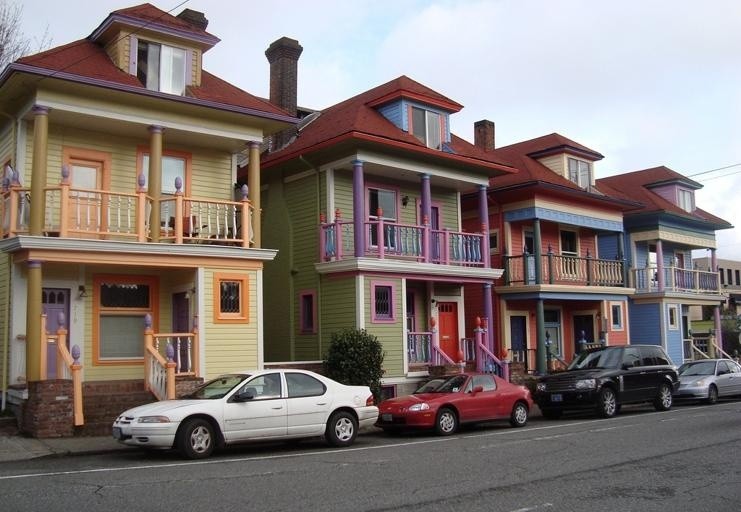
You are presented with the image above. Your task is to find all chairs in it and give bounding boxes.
[168,210,242,247]
[246,387,257,395]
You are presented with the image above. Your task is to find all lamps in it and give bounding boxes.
[402,195,409,207]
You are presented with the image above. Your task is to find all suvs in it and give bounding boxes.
[533,344,681,418]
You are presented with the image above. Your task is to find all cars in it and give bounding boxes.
[112,369,380,459]
[374,372,534,437]
[672,359,741,405]
